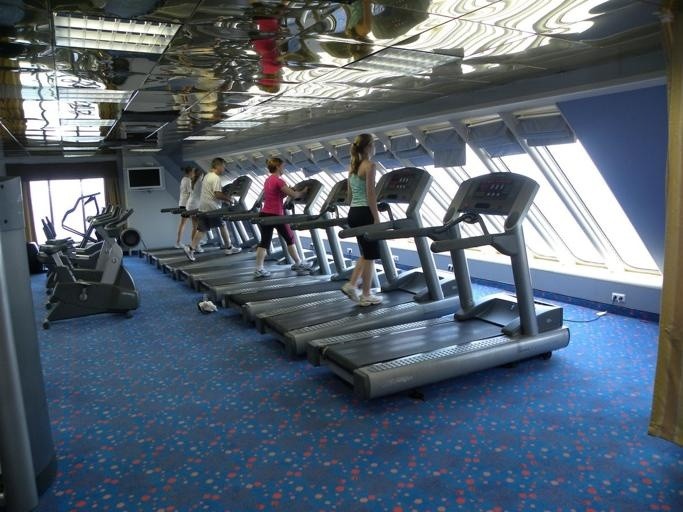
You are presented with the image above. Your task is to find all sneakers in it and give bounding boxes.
[361,294,383,306]
[342,283,360,301]
[255,270,270,277]
[296,261,314,271]
[174,241,205,261]
[224,245,242,254]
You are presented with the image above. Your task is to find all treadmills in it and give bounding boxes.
[307,173,571,401]
[140,176,353,319]
[254,168,466,357]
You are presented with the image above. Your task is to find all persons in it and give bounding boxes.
[251,156,314,279]
[157,0,393,133]
[187,168,207,257]
[175,162,192,251]
[184,158,241,261]
[340,133,384,307]
